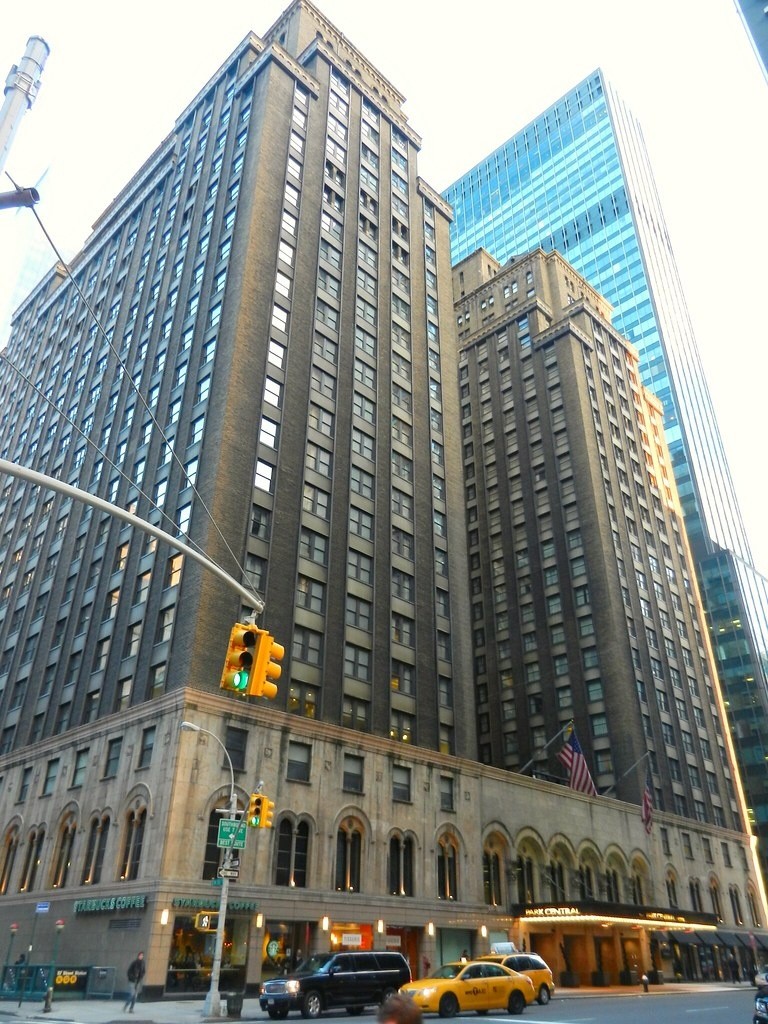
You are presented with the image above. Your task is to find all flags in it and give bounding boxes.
[554,727,598,796]
[642,754,653,834]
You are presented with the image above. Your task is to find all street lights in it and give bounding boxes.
[181,721,238,1018]
[43,920,64,1012]
[0,924,18,991]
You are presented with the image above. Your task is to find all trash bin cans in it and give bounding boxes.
[227,995,243,1018]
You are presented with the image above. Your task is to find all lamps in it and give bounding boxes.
[161,908,487,938]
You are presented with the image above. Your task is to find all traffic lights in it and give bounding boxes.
[247,635,284,701]
[220,624,253,695]
[194,913,212,931]
[248,795,262,828]
[259,797,276,829]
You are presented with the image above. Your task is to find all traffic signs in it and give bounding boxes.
[217,867,239,878]
[223,860,241,868]
[217,819,247,848]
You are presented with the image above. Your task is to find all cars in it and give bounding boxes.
[751,965,768,1024]
[398,959,536,1018]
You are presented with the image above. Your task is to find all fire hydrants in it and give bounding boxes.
[642,975,650,992]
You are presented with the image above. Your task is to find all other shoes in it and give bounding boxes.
[123,1007,134,1014]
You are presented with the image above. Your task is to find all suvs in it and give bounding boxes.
[258,950,412,1020]
[473,942,555,1004]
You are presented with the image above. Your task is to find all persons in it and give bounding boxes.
[460,949,469,961]
[123,951,146,1013]
[728,953,741,984]
[166,945,291,991]
[291,949,304,972]
[15,954,28,990]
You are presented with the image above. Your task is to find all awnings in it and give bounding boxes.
[651,929,768,951]
